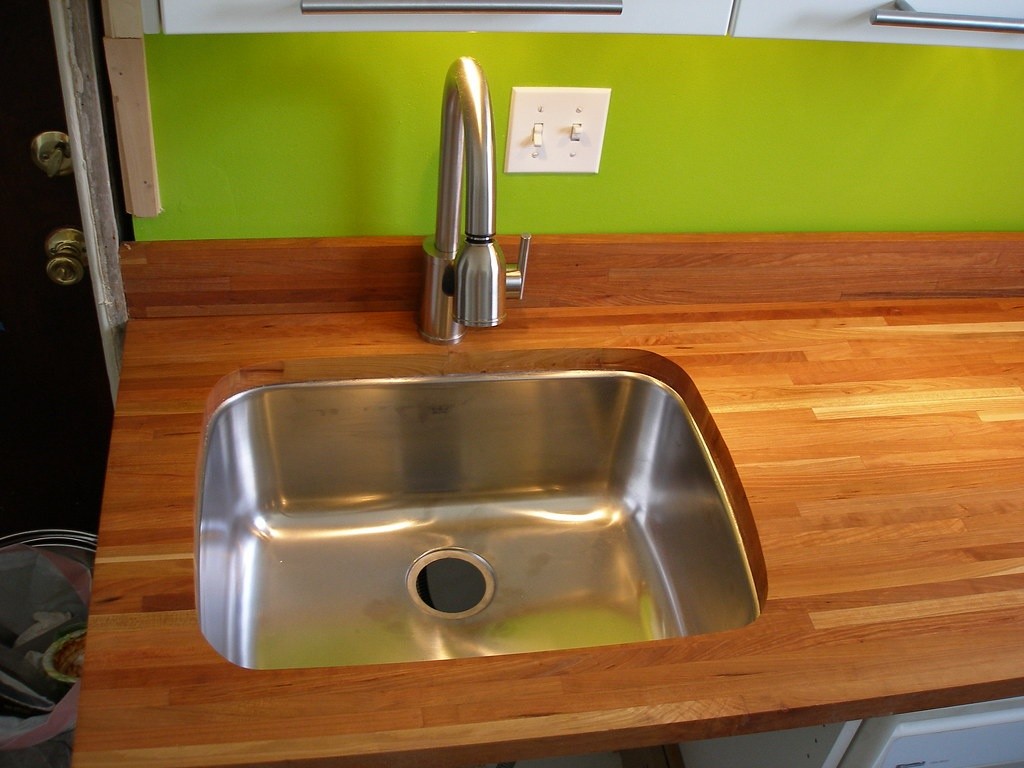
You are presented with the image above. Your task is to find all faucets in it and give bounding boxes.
[419,56,532,347]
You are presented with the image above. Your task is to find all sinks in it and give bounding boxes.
[193,345,769,671]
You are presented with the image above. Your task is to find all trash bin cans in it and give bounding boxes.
[0,529,98,768]
